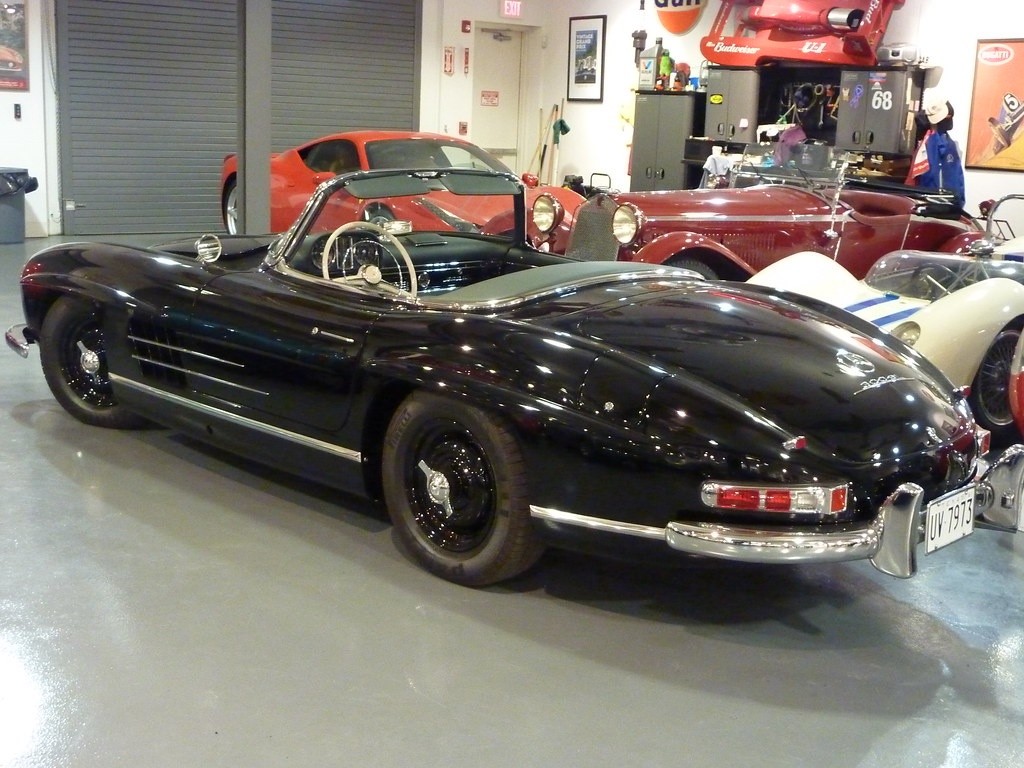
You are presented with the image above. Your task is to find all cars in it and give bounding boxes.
[221,131,590,254]
[564,143,985,280]
[4,167,994,591]
[743,232,1024,533]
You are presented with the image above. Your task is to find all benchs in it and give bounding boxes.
[436,259,684,301]
[818,188,916,215]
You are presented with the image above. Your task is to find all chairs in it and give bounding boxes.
[308,141,446,175]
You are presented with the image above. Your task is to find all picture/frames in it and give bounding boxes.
[567,14,608,100]
[963,37,1024,171]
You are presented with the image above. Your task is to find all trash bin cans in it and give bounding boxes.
[0,167,28,245]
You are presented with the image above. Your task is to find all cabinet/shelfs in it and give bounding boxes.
[836,66,927,158]
[706,63,770,144]
[629,88,704,193]
